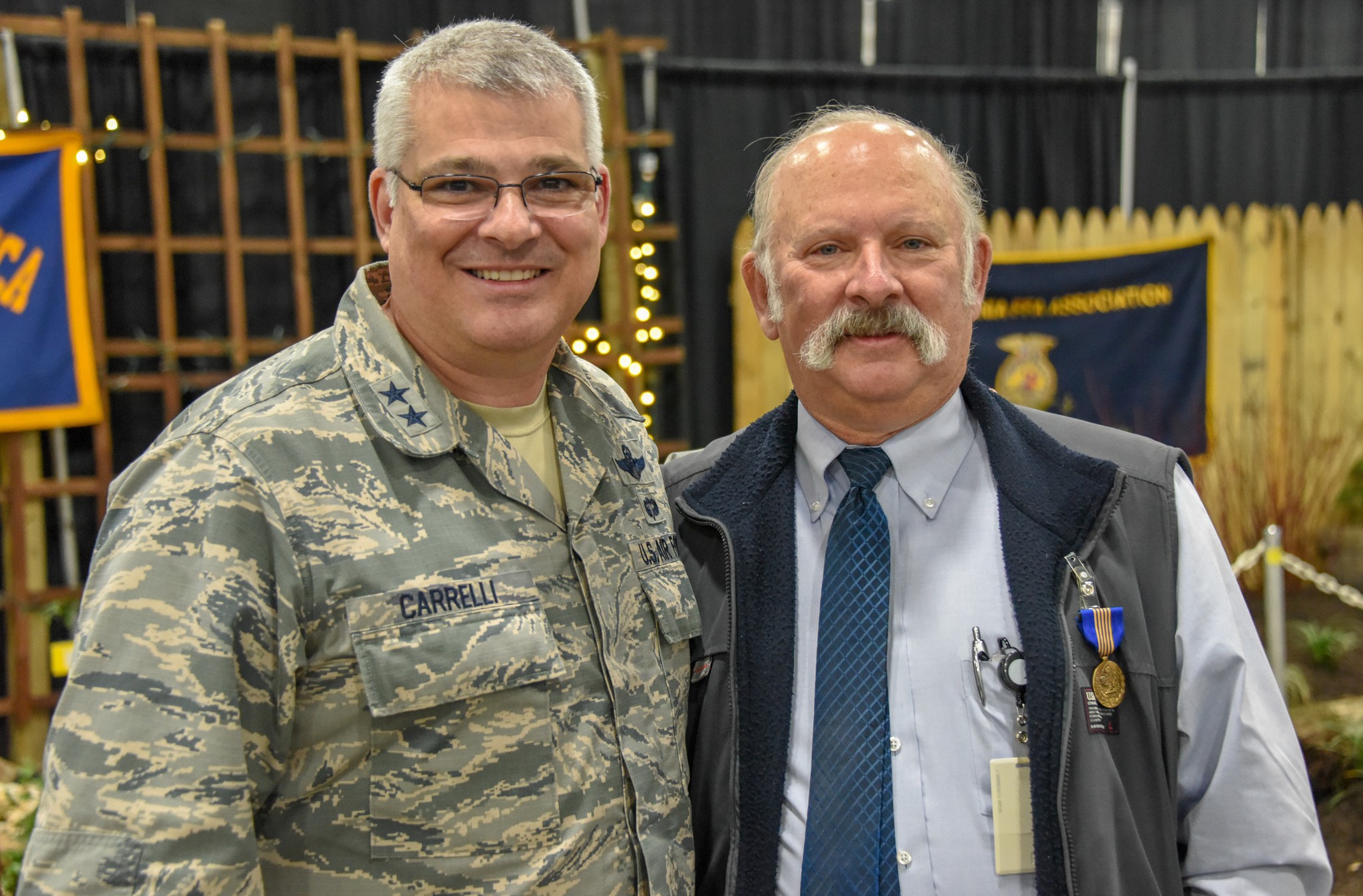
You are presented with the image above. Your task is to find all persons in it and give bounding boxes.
[655,100,1343,896]
[10,17,707,896]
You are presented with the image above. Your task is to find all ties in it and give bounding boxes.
[798,445,902,896]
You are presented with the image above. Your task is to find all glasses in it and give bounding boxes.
[385,167,604,218]
